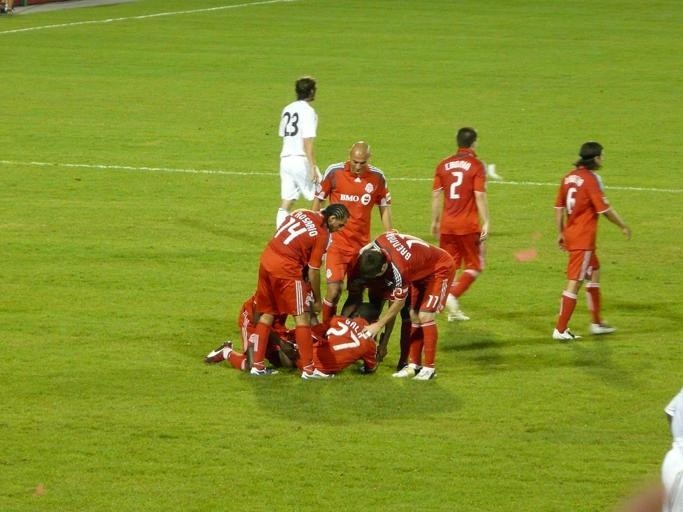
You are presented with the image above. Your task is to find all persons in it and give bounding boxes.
[429,127,491,324]
[205,204,457,383]
[309,140,394,324]
[612,384,683,512]
[274,76,325,233]
[551,139,632,341]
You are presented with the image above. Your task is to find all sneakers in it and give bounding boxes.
[203,341,232,363]
[552,327,580,340]
[413,367,435,381]
[300,368,334,381]
[447,312,469,322]
[392,363,421,378]
[250,367,278,376]
[591,323,614,334]
